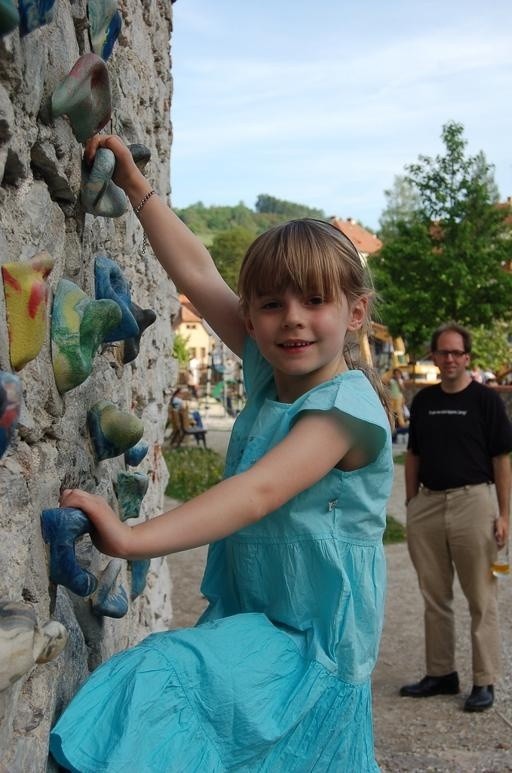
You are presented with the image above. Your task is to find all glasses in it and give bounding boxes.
[434,349,467,357]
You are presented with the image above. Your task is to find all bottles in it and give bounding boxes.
[492,540,510,576]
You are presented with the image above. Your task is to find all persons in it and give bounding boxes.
[399,327,512,713]
[49,131,395,773]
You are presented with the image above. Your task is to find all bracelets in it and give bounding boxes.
[135,190,156,217]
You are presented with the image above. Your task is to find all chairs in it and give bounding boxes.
[167,408,200,446]
[176,406,207,449]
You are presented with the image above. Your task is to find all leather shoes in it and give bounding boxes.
[464,683,493,712]
[399,670,459,697]
